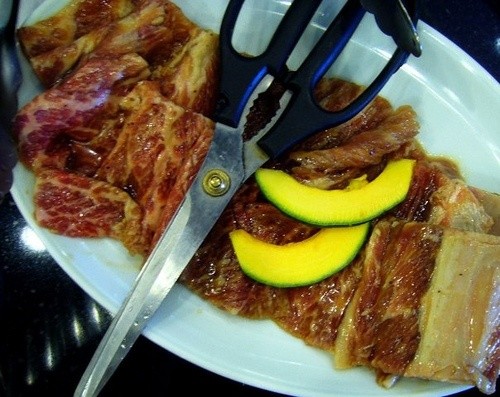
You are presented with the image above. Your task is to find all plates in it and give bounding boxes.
[8,0,499,395]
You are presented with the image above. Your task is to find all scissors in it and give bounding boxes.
[73,0,422,397]
